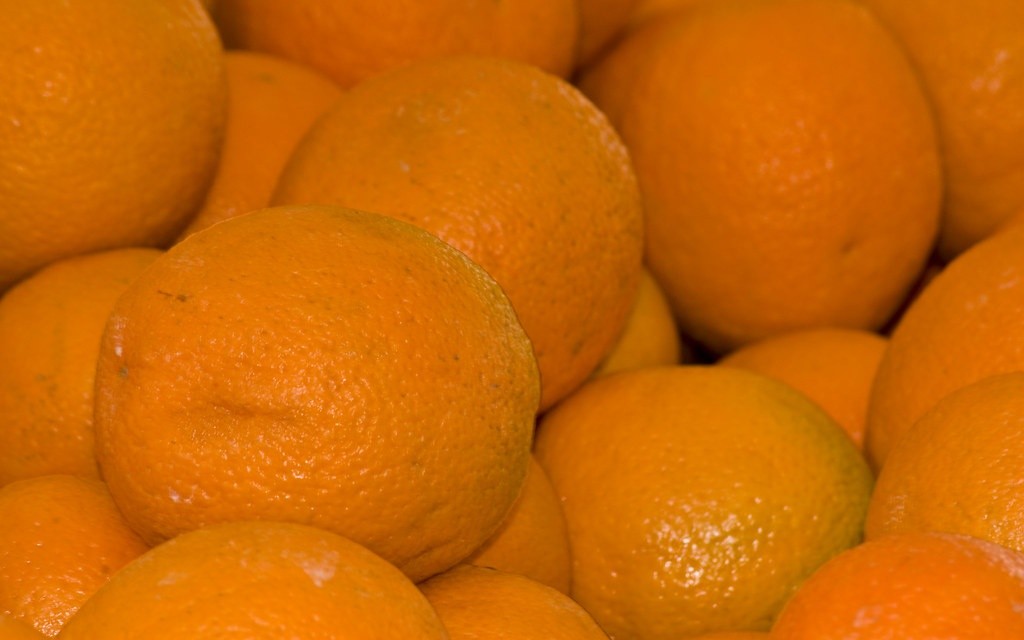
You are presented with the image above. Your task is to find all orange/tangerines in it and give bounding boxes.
[0,0,1024,640]
